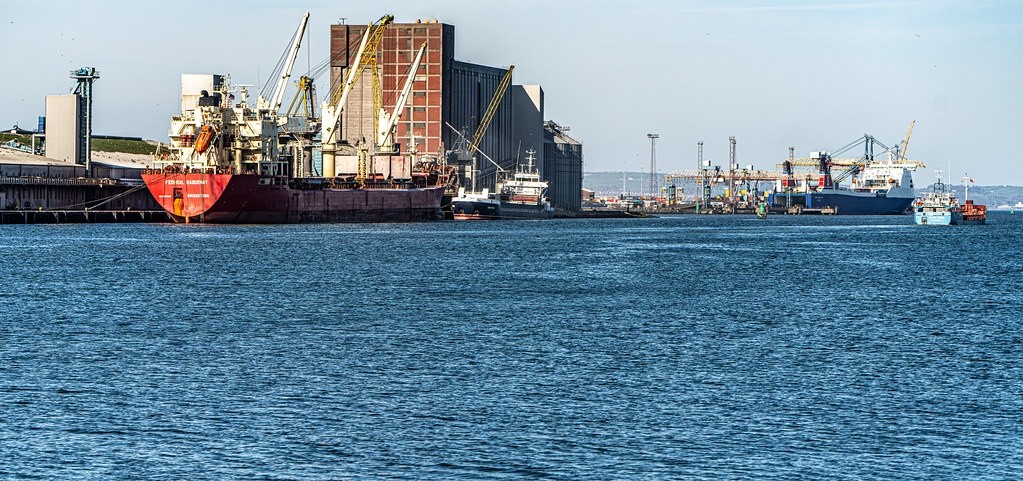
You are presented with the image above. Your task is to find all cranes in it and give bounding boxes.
[777,119,927,169]
[466,63,517,156]
[292,11,427,181]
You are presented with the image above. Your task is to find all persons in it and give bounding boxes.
[146,165,255,174]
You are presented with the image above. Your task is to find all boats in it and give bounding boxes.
[194,125,215,152]
[451,140,555,221]
[139,74,447,223]
[910,161,987,226]
[767,153,918,216]
